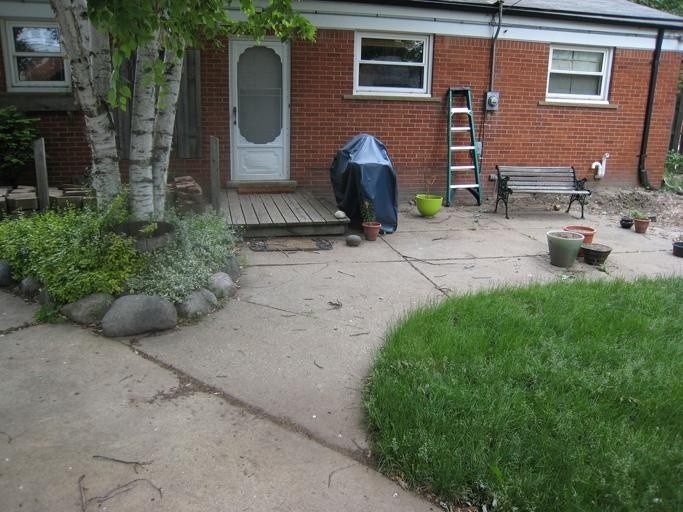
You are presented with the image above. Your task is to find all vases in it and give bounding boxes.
[546,226,612,268]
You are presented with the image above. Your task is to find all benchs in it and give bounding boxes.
[495,165,591,219]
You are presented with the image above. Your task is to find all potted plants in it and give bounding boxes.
[416,175,443,218]
[632,209,651,233]
[620,216,633,229]
[360,199,381,241]
[672,234,683,257]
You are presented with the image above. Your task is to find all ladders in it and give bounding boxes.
[447,87,482,206]
